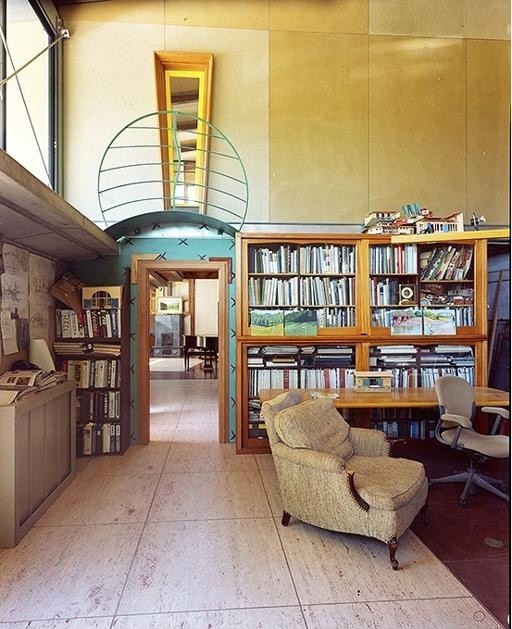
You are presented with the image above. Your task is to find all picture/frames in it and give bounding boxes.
[156,296,183,314]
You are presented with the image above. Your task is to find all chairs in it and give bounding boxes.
[260,389,429,570]
[427,376,510,507]
[183,336,218,368]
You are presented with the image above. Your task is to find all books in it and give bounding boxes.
[369,408,440,440]
[52,302,122,458]
[370,307,474,336]
[353,345,475,392]
[419,245,473,305]
[248,399,268,439]
[0,363,68,408]
[248,307,356,337]
[247,245,355,275]
[248,277,355,306]
[247,345,356,398]
[369,245,417,305]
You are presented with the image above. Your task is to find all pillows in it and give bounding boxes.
[274,398,354,461]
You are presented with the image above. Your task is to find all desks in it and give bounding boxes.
[0,379,76,548]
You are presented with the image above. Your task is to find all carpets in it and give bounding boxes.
[148,358,204,371]
[386,437,509,629]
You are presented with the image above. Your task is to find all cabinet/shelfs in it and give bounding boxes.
[55,267,130,456]
[235,231,487,453]
